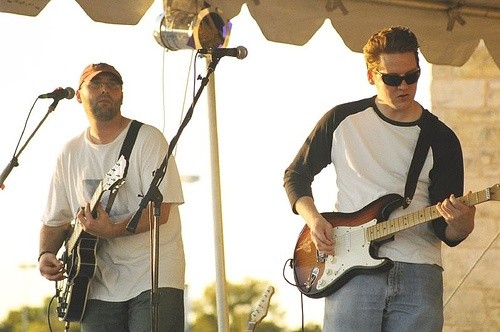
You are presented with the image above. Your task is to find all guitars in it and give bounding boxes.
[246,286,275,332]
[47,153,129,332]
[282,184,497,298]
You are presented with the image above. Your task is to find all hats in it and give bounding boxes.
[78,62,122,88]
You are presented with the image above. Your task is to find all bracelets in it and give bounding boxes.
[38,250,52,262]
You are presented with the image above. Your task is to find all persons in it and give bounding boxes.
[283,26,476,332]
[38,63,185,332]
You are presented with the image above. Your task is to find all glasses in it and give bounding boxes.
[372,65,421,87]
[86,80,121,90]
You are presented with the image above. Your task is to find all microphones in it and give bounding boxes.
[198,46,248,59]
[39,87,75,99]
